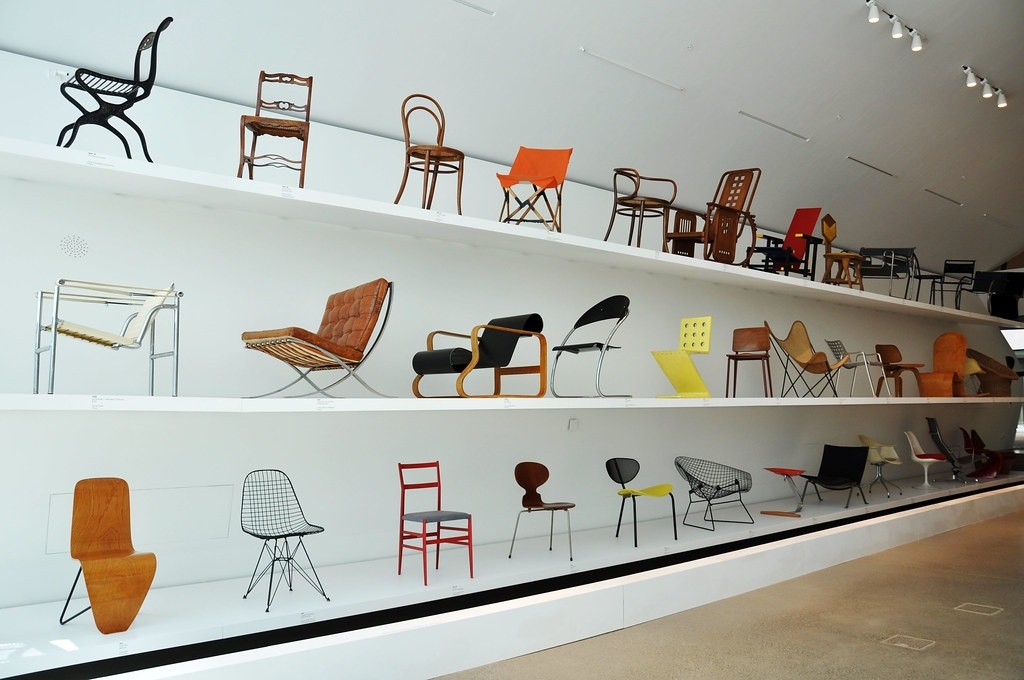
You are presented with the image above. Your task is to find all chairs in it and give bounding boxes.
[57,17,173,162]
[33,279,183,394]
[605,458,677,548]
[412,313,547,398]
[663,168,762,267]
[509,461,576,561]
[761,417,1024,517]
[726,320,1019,397]
[60,477,157,635]
[605,168,676,253]
[674,455,754,531]
[822,213,1024,317]
[398,461,473,586]
[551,294,630,397]
[237,70,313,189]
[393,94,465,216]
[746,207,823,281]
[240,469,331,612]
[242,278,399,400]
[496,146,574,232]
[651,316,712,397]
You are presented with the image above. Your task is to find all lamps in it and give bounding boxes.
[865,0,926,51]
[963,65,1007,108]
